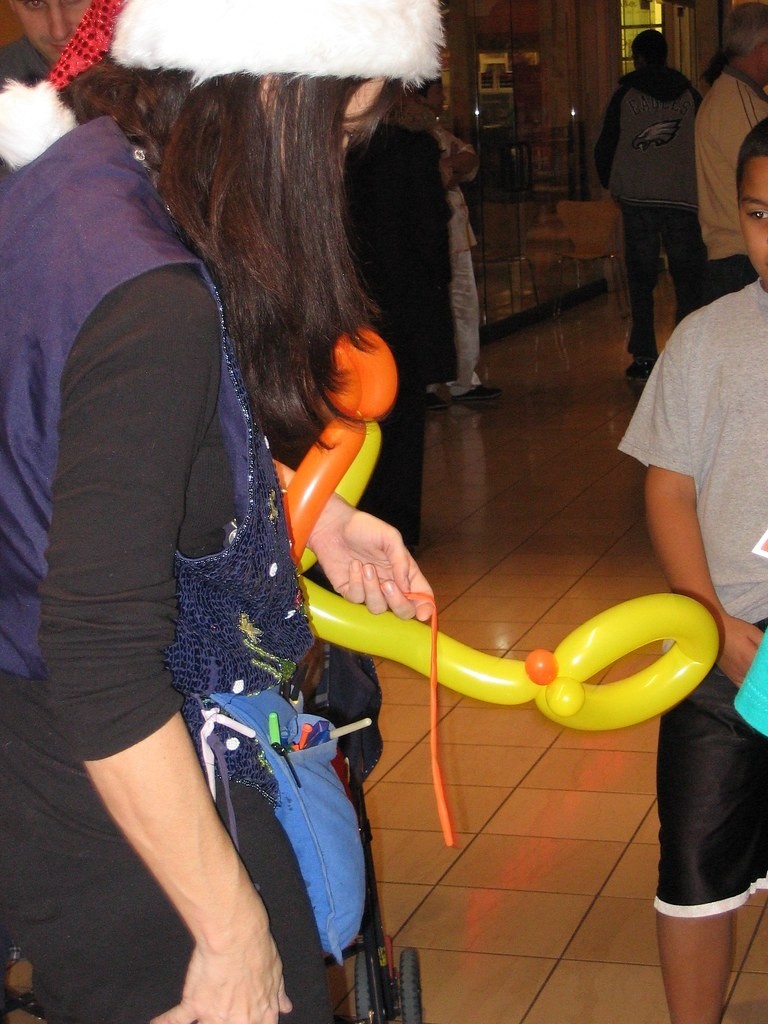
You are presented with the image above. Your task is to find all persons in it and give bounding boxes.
[0,0,768,1024]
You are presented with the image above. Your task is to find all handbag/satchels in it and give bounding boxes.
[209,688,366,964]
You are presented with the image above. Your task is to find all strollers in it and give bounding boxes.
[297,629,424,1024]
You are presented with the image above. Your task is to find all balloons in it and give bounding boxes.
[268,329,398,567]
[293,418,718,732]
[397,593,455,848]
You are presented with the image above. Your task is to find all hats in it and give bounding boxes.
[0,0,446,172]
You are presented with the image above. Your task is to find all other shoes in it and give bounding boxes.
[625,358,656,382]
[451,385,503,400]
[425,392,449,409]
